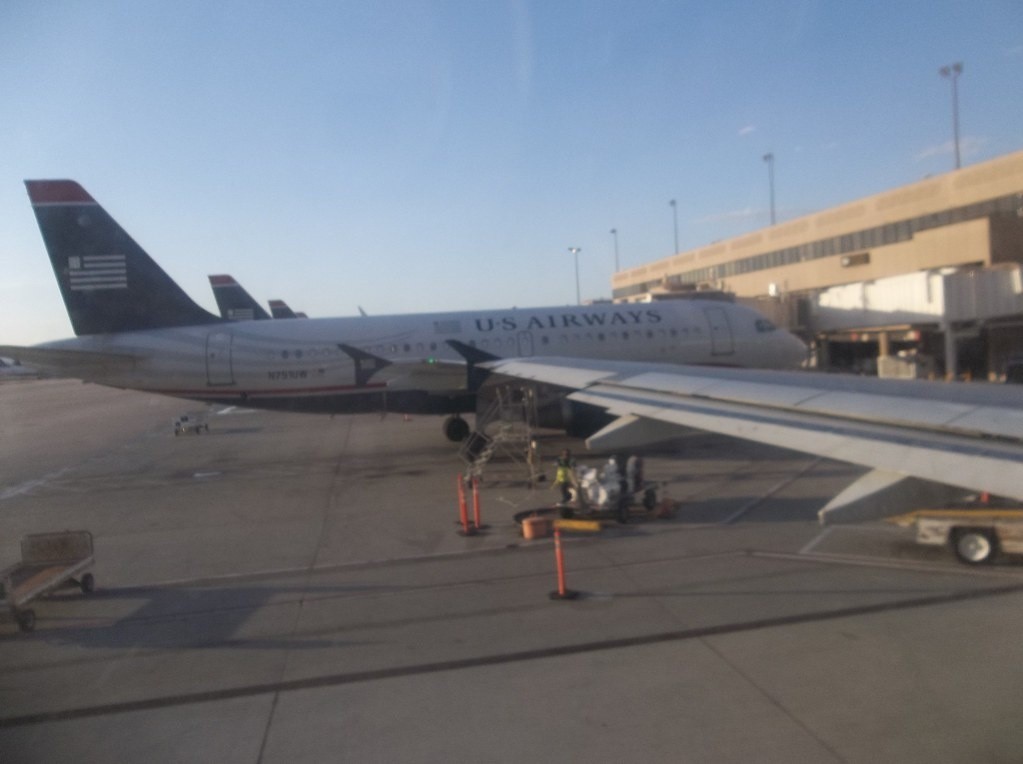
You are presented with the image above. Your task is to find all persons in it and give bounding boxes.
[550,449,578,503]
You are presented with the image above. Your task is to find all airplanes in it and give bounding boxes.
[1,177,1021,529]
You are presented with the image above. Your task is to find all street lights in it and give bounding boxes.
[758,150,778,228]
[940,62,964,174]
[608,229,620,272]
[567,247,581,307]
[668,199,678,258]
